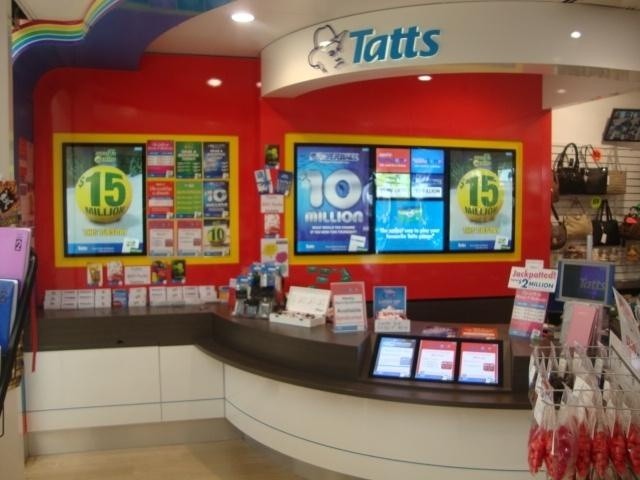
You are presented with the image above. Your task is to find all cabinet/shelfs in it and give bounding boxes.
[0,250,38,413]
[551,144,640,282]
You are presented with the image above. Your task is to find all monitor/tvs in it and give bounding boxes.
[368,334,505,390]
[553,258,614,308]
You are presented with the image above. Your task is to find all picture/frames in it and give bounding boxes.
[603,106,640,142]
